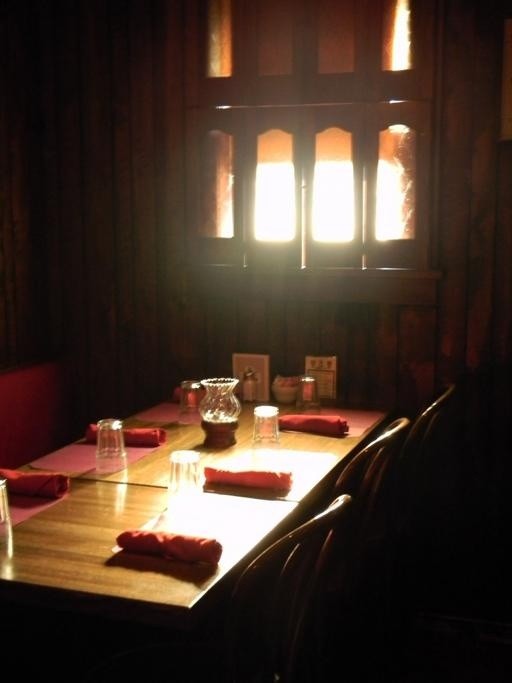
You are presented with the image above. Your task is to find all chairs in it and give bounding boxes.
[398,384,455,476]
[88,494,352,683]
[331,417,408,505]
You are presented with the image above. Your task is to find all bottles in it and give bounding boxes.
[92,417,128,474]
[297,376,319,402]
[241,368,256,403]
[175,380,202,414]
[246,403,281,444]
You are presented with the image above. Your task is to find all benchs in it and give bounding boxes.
[0,359,78,468]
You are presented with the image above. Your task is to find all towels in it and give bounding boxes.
[173,388,204,403]
[87,424,167,445]
[206,466,291,489]
[117,532,222,565]
[278,415,350,436]
[0,467,69,498]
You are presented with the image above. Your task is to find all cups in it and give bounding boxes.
[167,451,204,516]
[0,479,15,546]
